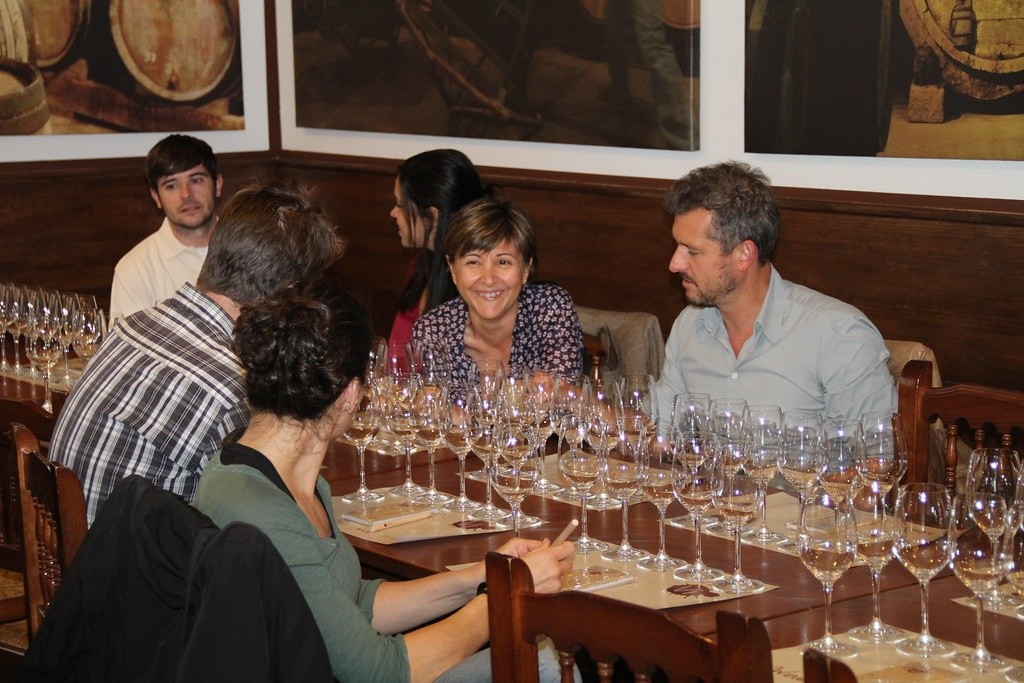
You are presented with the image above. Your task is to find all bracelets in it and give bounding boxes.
[477,581,488,595]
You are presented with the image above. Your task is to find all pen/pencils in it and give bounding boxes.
[551,519,579,547]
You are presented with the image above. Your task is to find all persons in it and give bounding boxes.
[598,1,700,150]
[189,279,578,683]
[108,133,224,334]
[414,197,583,410]
[388,148,494,387]
[535,159,898,483]
[47,172,346,529]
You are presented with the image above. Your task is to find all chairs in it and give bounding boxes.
[482,549,778,681]
[572,303,663,406]
[9,423,91,651]
[898,359,1024,490]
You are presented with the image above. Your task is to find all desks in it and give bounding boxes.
[1,285,1024,682]
